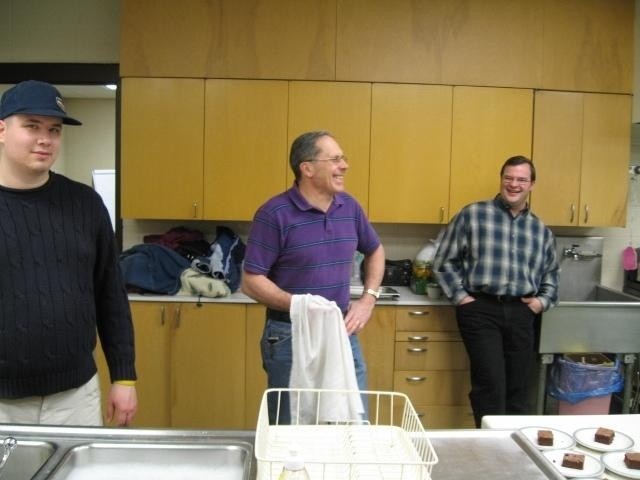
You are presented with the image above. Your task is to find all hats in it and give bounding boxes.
[0,80,82,125]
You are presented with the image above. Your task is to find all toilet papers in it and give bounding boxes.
[599,237,628,291]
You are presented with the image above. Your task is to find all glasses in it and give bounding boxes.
[503,176,531,183]
[302,155,345,162]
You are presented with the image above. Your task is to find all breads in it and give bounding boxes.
[537,430,554,446]
[595,426,615,444]
[562,453,585,470]
[625,452,639,468]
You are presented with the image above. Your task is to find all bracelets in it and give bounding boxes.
[111,380,137,386]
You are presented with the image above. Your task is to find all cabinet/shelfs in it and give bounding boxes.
[449,86,534,224]
[91,302,245,430]
[121,77,204,220]
[245,304,395,430]
[529,90,634,227]
[204,79,288,221]
[395,306,478,428]
[368,83,451,224]
[288,80,369,224]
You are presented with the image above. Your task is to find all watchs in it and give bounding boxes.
[363,288,380,300]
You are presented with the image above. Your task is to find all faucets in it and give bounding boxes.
[564,245,579,261]
[0,437,17,474]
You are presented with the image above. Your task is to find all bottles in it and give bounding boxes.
[410,263,438,294]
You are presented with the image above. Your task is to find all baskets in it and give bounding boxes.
[359,259,413,286]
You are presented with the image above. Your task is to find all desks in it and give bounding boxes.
[481,414,640,479]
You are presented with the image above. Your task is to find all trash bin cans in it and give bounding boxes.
[555,352,619,415]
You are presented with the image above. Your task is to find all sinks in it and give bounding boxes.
[0,440,57,480]
[46,441,252,480]
[538,284,640,355]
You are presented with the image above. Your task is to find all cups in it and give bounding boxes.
[426,284,443,299]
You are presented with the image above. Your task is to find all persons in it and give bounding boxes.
[433,155,560,429]
[241,130,386,426]
[0,79,138,427]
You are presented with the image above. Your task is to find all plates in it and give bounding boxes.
[520,427,640,480]
